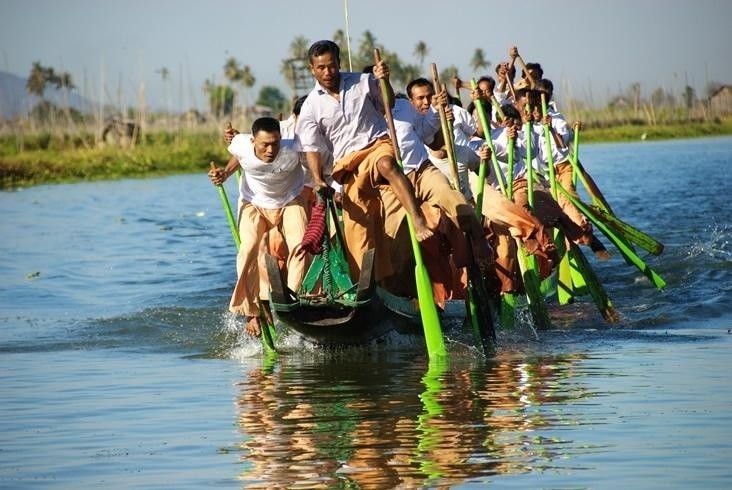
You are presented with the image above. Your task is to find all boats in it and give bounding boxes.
[264,248,557,349]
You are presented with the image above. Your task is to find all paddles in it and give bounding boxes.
[210,140,278,356]
[373,45,448,358]
[430,46,667,344]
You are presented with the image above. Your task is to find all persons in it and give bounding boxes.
[295,40,433,287]
[362,44,595,310]
[208,96,344,337]
